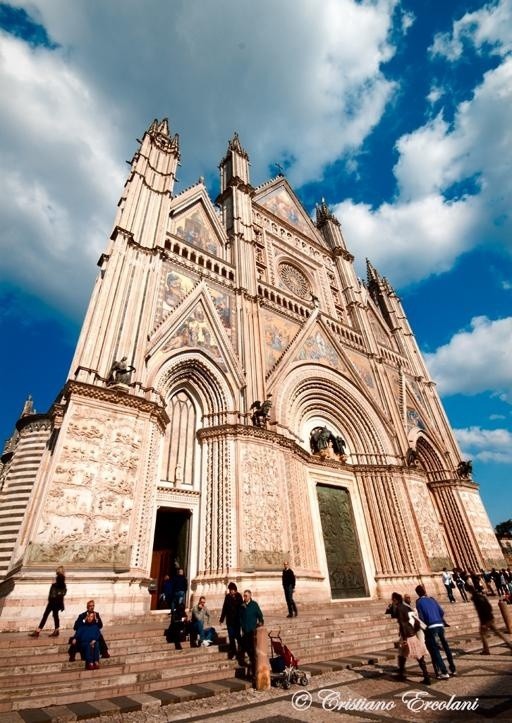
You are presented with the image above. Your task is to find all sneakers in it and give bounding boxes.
[452,670,458,676]
[436,673,450,679]
[201,639,213,647]
[85,661,100,670]
[420,679,431,685]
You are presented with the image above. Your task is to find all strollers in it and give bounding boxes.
[267,629,310,689]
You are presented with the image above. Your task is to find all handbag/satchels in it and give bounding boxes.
[268,655,287,673]
[449,578,456,588]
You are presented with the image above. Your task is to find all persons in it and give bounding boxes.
[28,565,68,637]
[439,564,512,603]
[112,356,130,384]
[168,567,188,615]
[156,574,171,607]
[72,611,101,671]
[66,599,110,661]
[282,560,299,618]
[167,603,201,650]
[403,594,413,607]
[415,585,456,678]
[388,592,431,685]
[192,597,215,647]
[238,588,264,666]
[465,584,512,654]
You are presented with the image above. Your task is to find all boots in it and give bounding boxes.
[27,630,40,637]
[48,629,59,637]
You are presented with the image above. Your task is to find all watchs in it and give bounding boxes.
[218,583,243,660]
